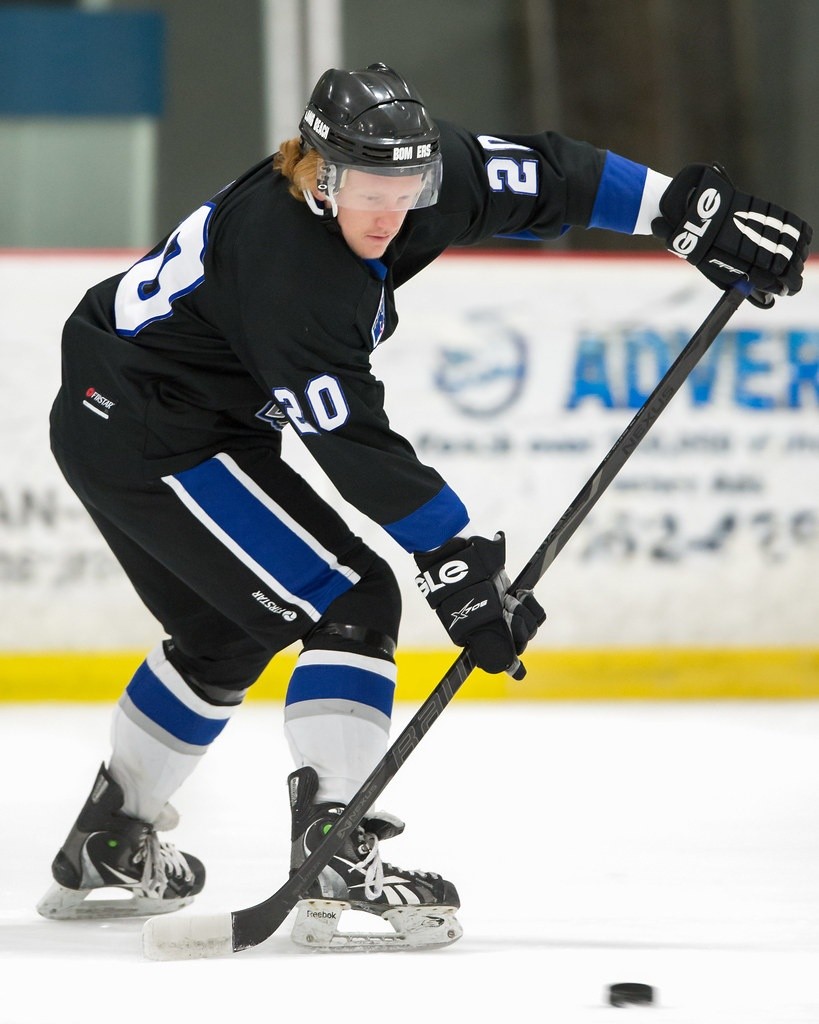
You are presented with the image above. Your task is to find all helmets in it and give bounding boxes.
[299,62,442,217]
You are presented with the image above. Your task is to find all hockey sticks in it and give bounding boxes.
[139,275,756,966]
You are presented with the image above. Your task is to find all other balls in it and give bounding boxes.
[608,983,654,1008]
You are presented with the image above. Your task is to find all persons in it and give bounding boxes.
[38,64,811,953]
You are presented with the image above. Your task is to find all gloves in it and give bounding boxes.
[651,161,813,309]
[414,530,546,681]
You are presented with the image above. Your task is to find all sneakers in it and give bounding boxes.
[288,766,463,953]
[36,761,206,920]
